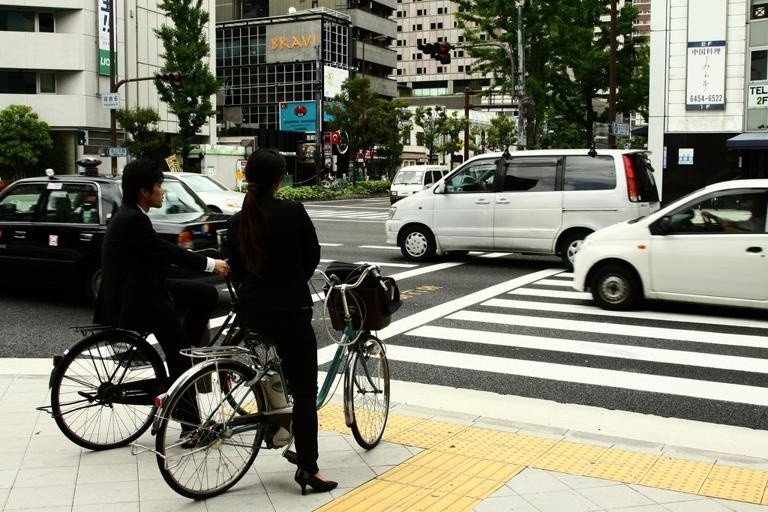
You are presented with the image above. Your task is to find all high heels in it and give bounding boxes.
[295,469,337,495]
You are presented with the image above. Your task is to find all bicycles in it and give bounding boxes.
[35,247,294,451]
[130,262,403,500]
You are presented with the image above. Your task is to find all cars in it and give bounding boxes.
[0,167,235,310]
[118,168,248,217]
[572,179,768,311]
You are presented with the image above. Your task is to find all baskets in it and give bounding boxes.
[322,280,392,331]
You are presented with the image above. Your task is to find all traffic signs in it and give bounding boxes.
[99,147,129,157]
[612,120,631,136]
[101,92,121,108]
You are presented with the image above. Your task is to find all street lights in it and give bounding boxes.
[362,36,386,181]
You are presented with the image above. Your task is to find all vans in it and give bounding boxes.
[390,164,450,206]
[385,141,661,272]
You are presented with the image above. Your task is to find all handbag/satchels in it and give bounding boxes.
[324,262,403,330]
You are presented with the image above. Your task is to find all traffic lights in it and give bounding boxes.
[434,52,451,64]
[77,131,86,145]
[155,71,183,82]
[413,40,451,53]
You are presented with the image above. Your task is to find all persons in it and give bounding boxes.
[699,197,766,232]
[92,158,230,449]
[229,145,338,494]
